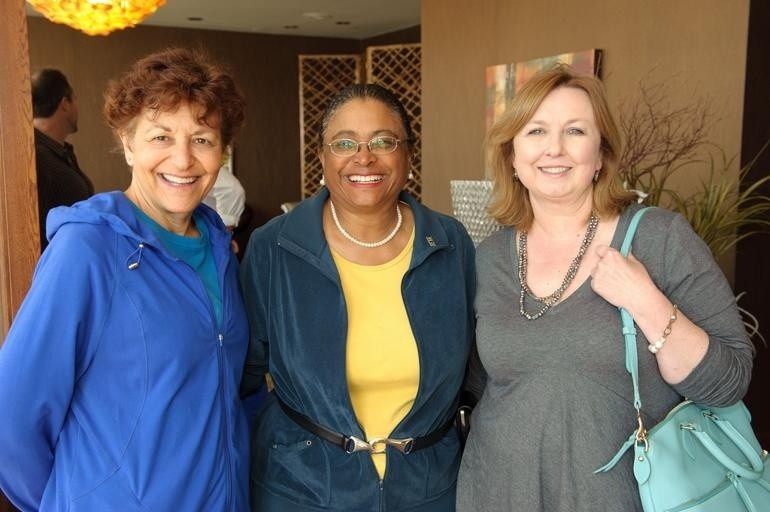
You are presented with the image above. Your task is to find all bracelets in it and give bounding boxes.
[644,303,680,353]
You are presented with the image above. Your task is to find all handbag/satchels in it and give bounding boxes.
[633,401,770,512]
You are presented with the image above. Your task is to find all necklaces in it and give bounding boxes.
[513,209,599,321]
[329,199,403,248]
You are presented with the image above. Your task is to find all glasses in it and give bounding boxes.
[319,135,411,156]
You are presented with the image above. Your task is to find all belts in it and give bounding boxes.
[272,388,459,456]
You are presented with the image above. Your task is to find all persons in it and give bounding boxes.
[0,47,261,512]
[447,63,753,512]
[221,79,491,511]
[27,66,95,240]
[193,157,249,257]
